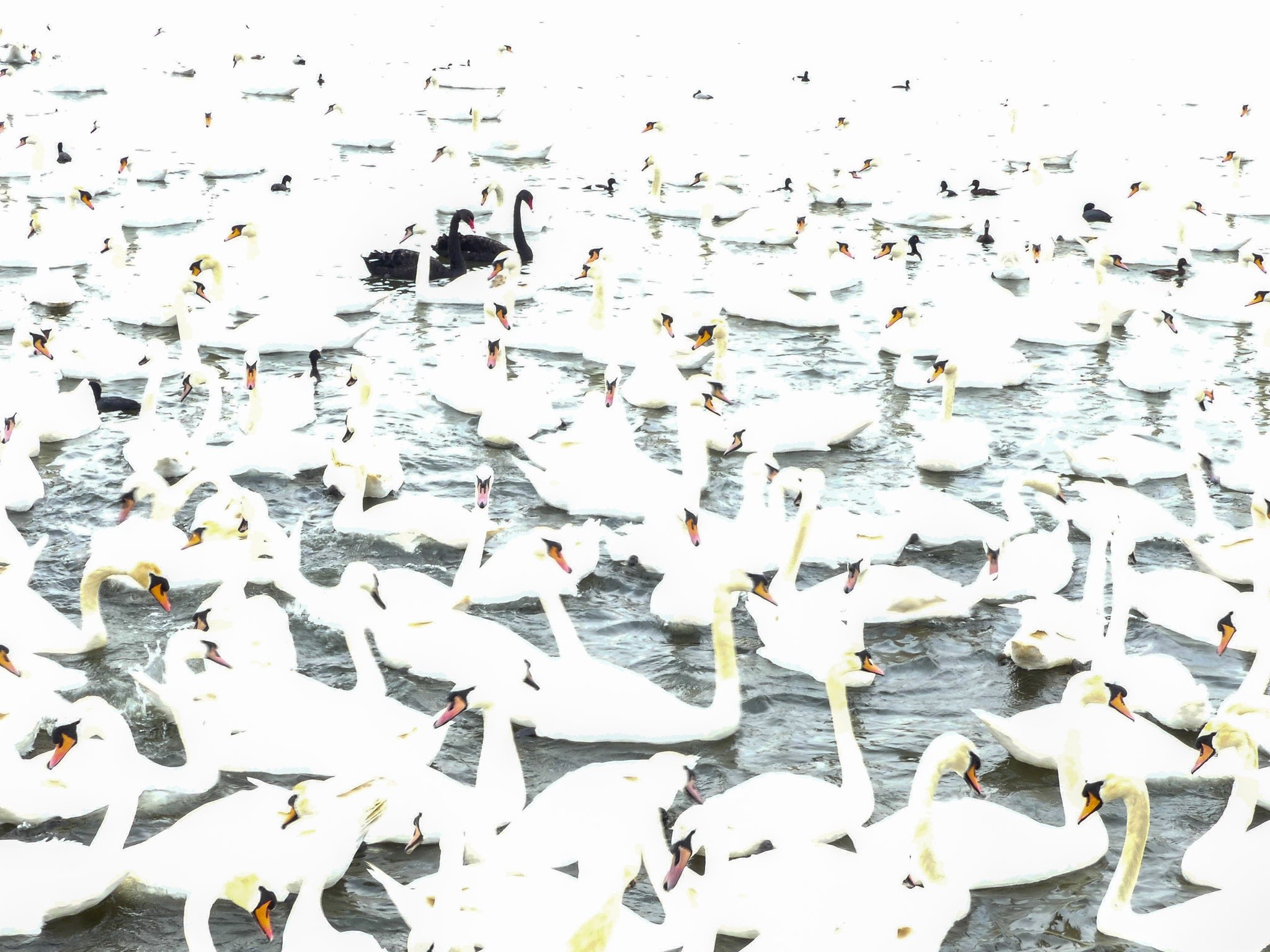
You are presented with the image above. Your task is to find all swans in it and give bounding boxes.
[0,0,1270,952]
